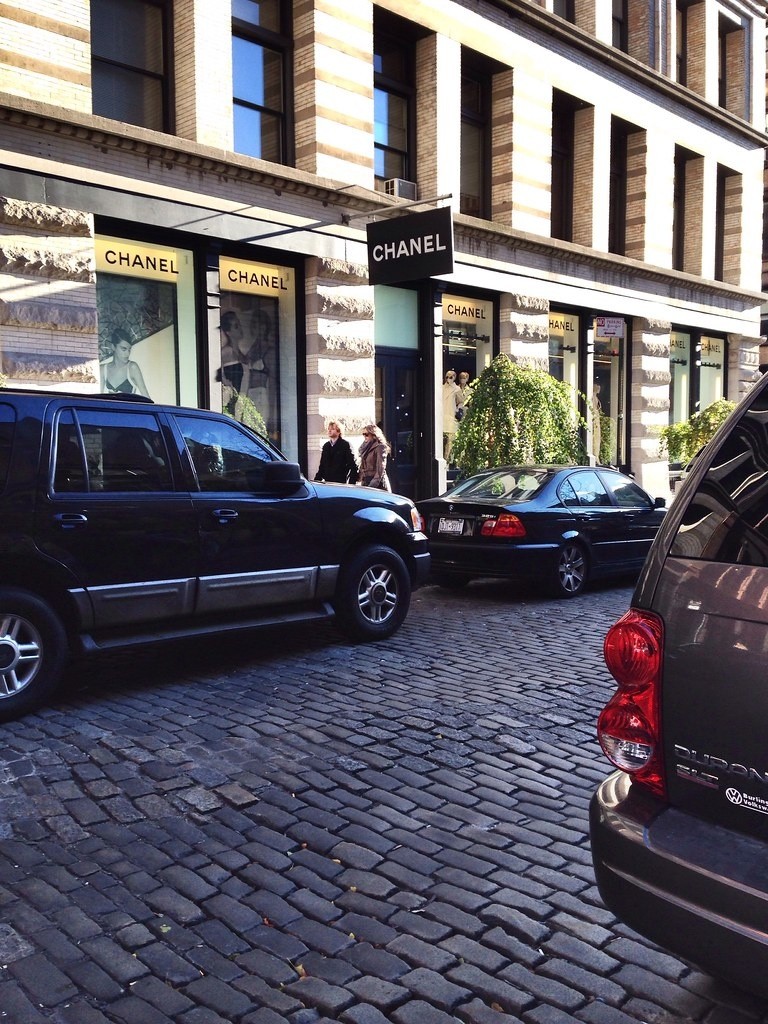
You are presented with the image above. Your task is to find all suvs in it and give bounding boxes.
[0,388,431,722]
[587,360,768,1005]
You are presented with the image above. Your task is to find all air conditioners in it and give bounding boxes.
[385,178,417,202]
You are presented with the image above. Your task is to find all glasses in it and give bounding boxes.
[446,376,453,379]
[363,433,372,436]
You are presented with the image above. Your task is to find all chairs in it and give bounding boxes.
[119,433,172,491]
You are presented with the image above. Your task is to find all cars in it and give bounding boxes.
[414,464,669,600]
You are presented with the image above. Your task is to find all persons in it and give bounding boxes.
[443,371,467,460]
[358,424,391,492]
[206,443,224,475]
[220,310,276,428]
[314,420,357,484]
[100,328,150,399]
[457,371,474,412]
[592,382,603,466]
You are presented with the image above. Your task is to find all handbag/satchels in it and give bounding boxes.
[362,475,387,490]
